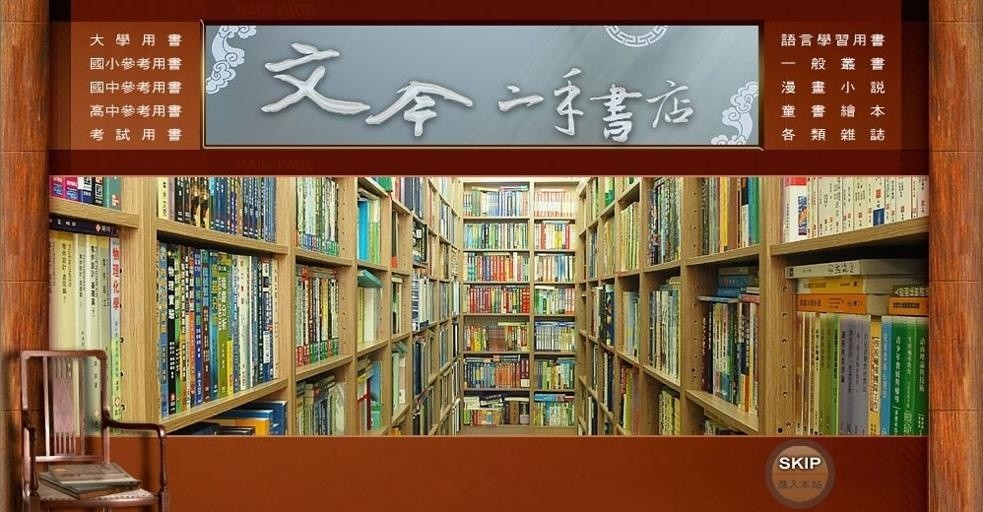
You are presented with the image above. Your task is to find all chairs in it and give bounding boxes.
[16,341,168,512]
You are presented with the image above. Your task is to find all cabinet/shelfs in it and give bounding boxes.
[47,170,464,437]
[462,176,583,438]
[572,174,935,439]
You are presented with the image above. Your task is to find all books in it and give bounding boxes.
[649,176,682,264]
[295,175,341,255]
[696,265,761,435]
[35,471,142,498]
[412,277,436,328]
[578,174,639,277]
[429,175,457,278]
[648,277,679,379]
[464,393,577,428]
[413,221,427,275]
[156,176,277,242]
[462,321,576,352]
[575,282,636,433]
[783,261,928,436]
[295,374,348,437]
[462,220,576,249]
[390,342,407,418]
[294,264,340,367]
[48,460,139,485]
[356,354,385,434]
[48,213,122,429]
[391,273,406,337]
[658,388,681,436]
[437,281,459,321]
[154,242,287,439]
[462,183,577,218]
[391,210,400,269]
[355,268,382,350]
[412,324,463,435]
[465,251,576,281]
[467,284,576,315]
[696,176,759,257]
[357,186,382,261]
[51,174,123,210]
[780,175,928,244]
[463,354,574,391]
[383,176,427,222]
